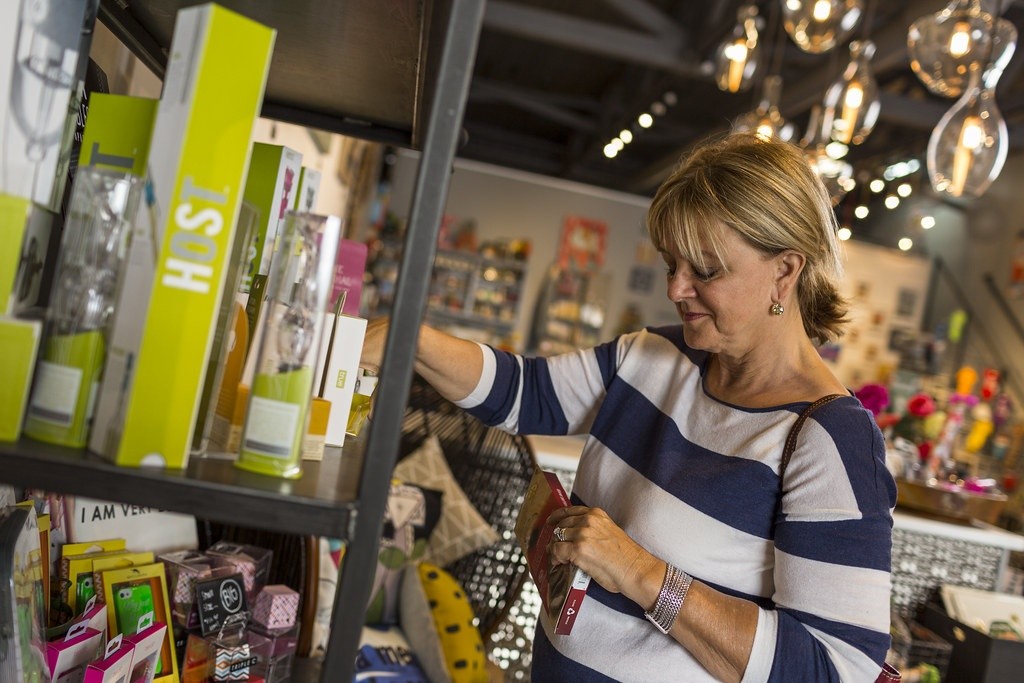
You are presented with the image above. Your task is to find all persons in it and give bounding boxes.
[360,132,896,683]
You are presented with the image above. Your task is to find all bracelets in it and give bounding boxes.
[646,564,693,635]
[554,527,566,541]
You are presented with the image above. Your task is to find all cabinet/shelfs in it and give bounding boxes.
[372,241,527,335]
[0,0,487,683]
[524,260,613,360]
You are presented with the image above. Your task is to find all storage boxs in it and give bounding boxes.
[514,465,591,635]
[0,3,369,480]
[916,601,1024,683]
[158,541,301,683]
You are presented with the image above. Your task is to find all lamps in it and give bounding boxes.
[713,0,1018,207]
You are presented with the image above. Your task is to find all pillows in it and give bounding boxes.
[308,434,493,683]
